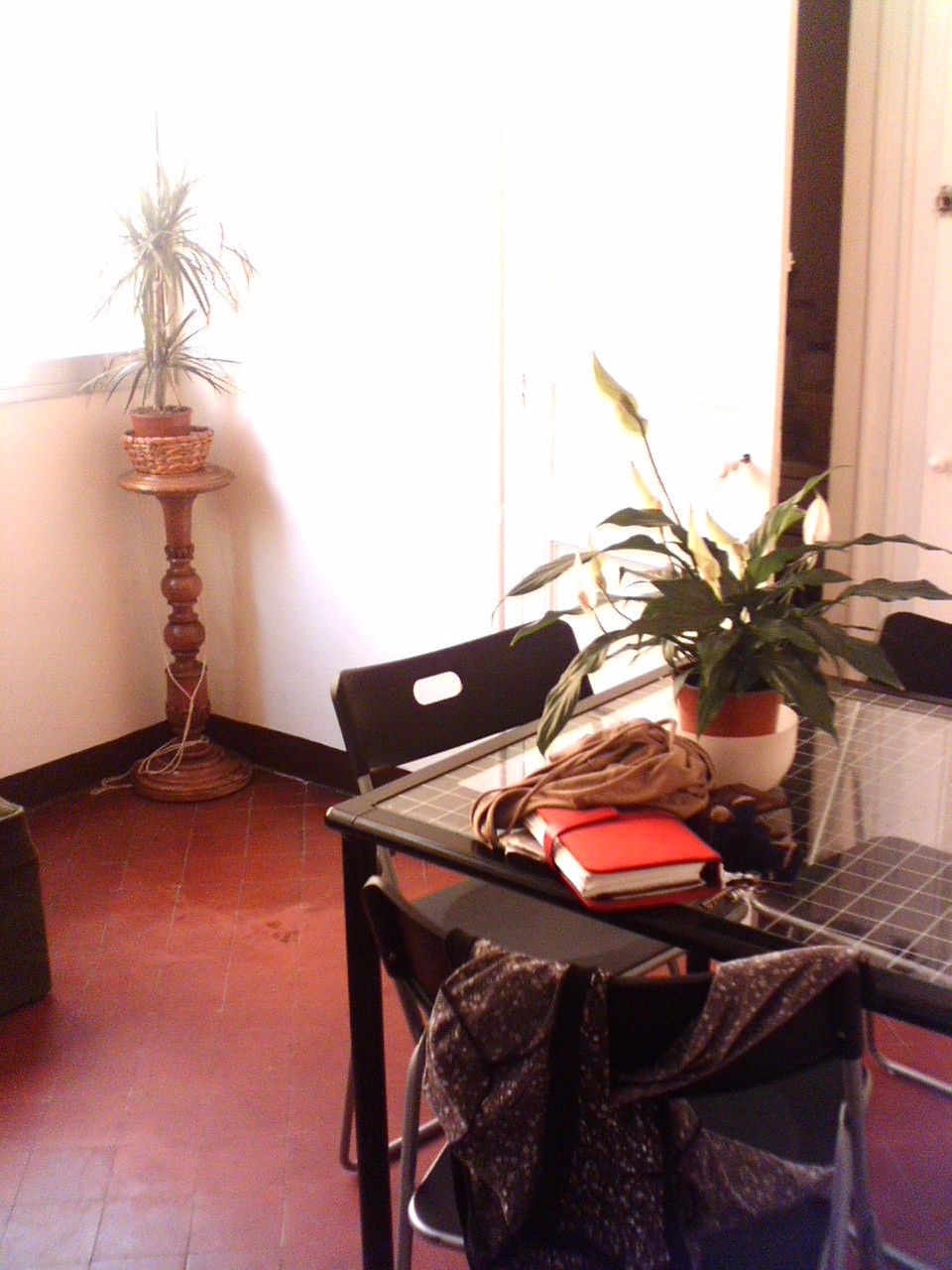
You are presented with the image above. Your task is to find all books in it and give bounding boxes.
[523,805,726,912]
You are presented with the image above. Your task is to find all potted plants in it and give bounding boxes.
[77,166,260,437]
[491,351,952,739]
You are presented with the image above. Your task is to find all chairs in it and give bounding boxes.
[360,874,880,1270]
[789,611,952,1270]
[331,616,684,1171]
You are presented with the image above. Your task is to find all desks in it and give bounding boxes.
[325,662,952,1270]
[120,463,255,801]
